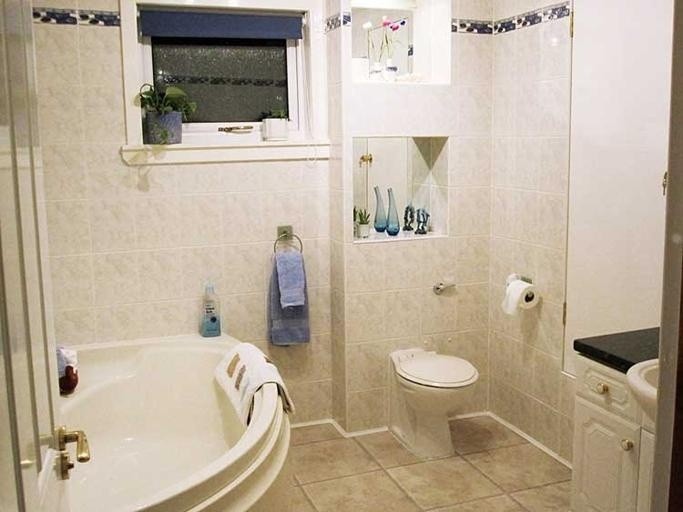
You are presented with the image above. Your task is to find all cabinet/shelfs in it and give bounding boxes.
[567,352,656,512]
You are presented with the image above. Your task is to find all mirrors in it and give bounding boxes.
[352,136,433,235]
[351,0,431,83]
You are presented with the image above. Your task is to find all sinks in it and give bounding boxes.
[627,358,660,424]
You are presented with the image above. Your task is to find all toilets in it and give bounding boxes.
[387,348,479,460]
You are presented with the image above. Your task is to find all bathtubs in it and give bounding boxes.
[56,331,291,512]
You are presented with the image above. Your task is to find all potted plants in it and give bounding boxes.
[139,79,197,145]
[353,206,358,236]
[261,109,292,142]
[356,209,370,238]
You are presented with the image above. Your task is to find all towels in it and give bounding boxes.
[266,250,310,347]
[213,342,295,428]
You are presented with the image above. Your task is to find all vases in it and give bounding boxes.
[385,188,400,236]
[373,186,386,232]
